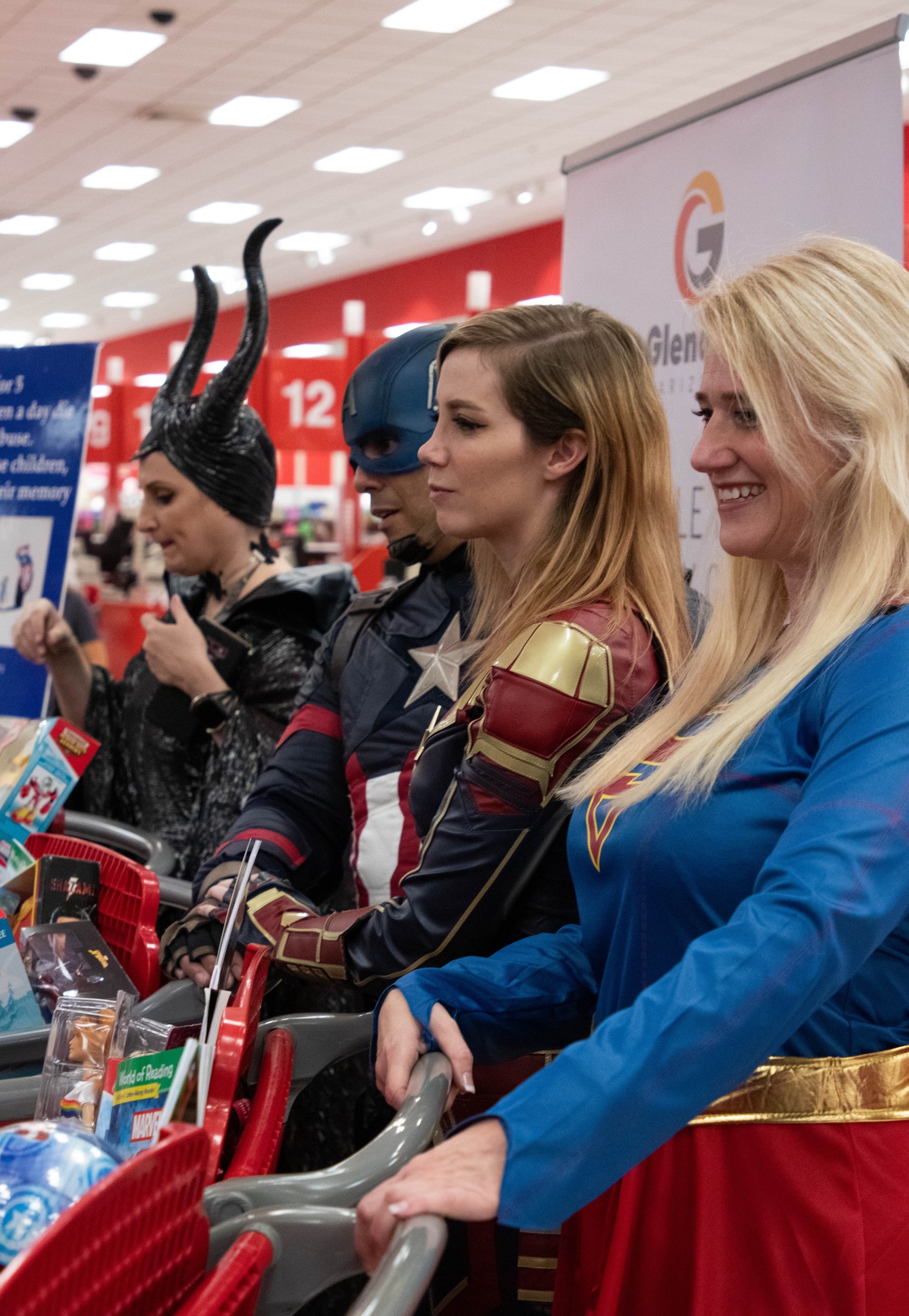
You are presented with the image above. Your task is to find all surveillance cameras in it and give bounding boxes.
[151,10,174,24]
[12,109,35,121]
[75,66,94,79]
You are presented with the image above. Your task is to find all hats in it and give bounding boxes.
[338,321,459,475]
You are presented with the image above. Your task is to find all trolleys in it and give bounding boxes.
[0,802,459,1315]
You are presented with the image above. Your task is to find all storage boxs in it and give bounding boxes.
[0,714,102,845]
[19,920,141,1013]
[0,854,102,949]
[32,989,137,1130]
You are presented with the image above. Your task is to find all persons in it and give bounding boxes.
[194,299,702,1316]
[15,213,383,1166]
[151,324,488,1166]
[354,239,908,1316]
